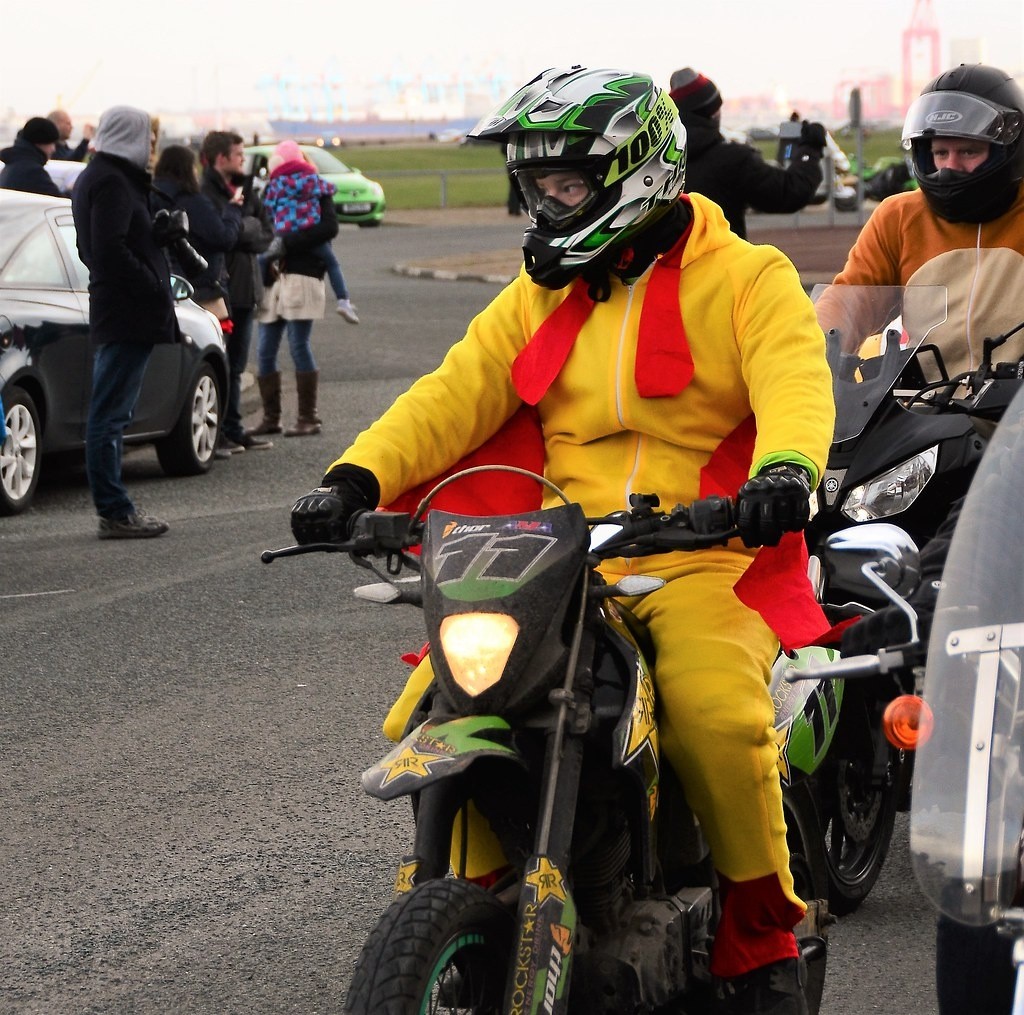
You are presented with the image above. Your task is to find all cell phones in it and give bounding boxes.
[779,122,803,138]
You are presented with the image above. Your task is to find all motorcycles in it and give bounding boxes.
[781,279,1024,924]
[766,125,921,202]
[256,463,875,1014]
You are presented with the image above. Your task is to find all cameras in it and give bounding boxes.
[153,210,208,277]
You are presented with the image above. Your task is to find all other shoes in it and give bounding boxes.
[231,435,273,450]
[219,438,248,453]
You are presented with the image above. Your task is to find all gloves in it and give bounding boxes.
[290,480,369,546]
[734,465,815,547]
[802,120,826,153]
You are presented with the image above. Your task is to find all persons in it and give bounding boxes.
[70,104,184,540]
[154,130,360,458]
[288,66,840,1015]
[668,67,828,241]
[47,111,94,162]
[815,62,1024,447]
[0,117,77,199]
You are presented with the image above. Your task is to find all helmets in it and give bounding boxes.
[912,64,1024,223]
[468,65,688,289]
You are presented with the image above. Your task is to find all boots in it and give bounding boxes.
[284,368,323,435]
[246,371,285,434]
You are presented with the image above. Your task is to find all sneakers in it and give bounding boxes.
[732,940,809,1015]
[98,507,170,536]
[337,298,360,325]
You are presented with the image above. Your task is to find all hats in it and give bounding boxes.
[273,139,304,165]
[669,67,722,118]
[22,117,60,146]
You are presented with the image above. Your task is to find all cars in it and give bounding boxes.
[745,124,784,140]
[317,132,343,148]
[459,132,501,148]
[241,143,386,229]
[0,188,236,515]
